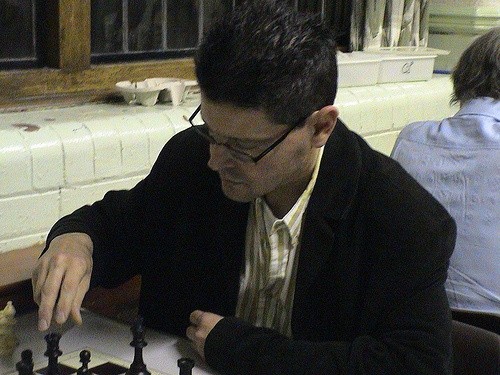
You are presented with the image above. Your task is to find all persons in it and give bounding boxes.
[31,4,458,375]
[388,25,500,337]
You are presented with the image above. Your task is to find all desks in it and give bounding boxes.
[0,305,214,375]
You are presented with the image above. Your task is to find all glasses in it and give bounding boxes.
[188,102,306,166]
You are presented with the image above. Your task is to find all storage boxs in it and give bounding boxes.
[354,50,438,85]
[336,52,384,88]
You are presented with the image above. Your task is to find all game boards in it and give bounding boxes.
[1,347,169,375]
